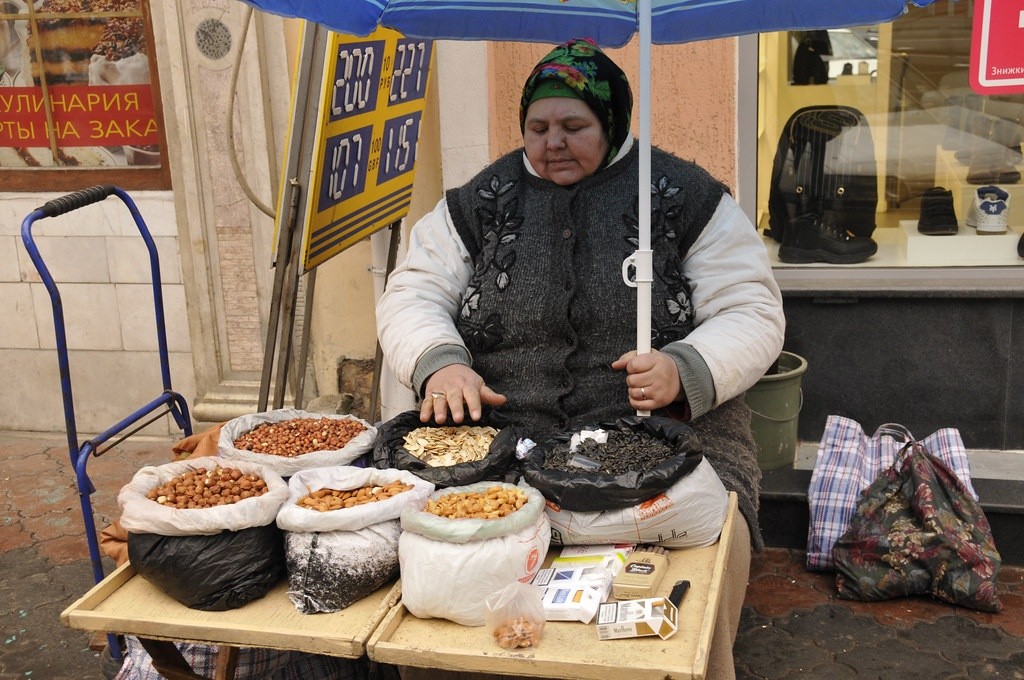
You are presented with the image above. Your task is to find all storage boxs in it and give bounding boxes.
[528,543,679,642]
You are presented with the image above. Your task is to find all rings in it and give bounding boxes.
[641,388,646,400]
[431,393,445,398]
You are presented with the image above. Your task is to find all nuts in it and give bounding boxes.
[143,466,268,510]
[234,416,371,458]
[421,485,527,521]
[294,479,415,513]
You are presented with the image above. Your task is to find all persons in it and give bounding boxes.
[375,38,785,555]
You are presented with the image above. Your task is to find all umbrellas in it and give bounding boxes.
[241,0,928,415]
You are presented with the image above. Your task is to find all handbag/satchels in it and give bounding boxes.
[806,414,979,571]
[832,441,1003,614]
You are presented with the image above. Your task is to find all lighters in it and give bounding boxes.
[669,579,690,607]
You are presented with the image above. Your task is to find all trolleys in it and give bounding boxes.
[19,183,209,679]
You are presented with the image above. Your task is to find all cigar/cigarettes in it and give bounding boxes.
[652,600,664,606]
[637,545,669,556]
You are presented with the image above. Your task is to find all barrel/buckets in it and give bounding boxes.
[743,351,808,471]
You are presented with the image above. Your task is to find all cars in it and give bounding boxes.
[791,27,879,81]
[863,28,878,47]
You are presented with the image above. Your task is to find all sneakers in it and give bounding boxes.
[918,186,959,235]
[778,214,878,264]
[965,186,1011,234]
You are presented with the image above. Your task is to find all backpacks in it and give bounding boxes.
[763,105,878,245]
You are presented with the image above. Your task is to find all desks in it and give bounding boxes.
[58,491,738,680]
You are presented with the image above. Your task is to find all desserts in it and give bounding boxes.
[23,0,151,87]
